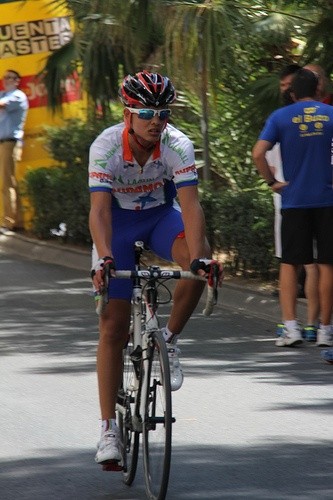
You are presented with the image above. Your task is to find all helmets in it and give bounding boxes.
[119,70,177,107]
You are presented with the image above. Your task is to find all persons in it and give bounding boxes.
[252,64,333,348]
[0,70,29,231]
[88,71,224,464]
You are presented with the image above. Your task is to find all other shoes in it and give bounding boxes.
[275,323,285,336]
[0,226,27,237]
[303,326,317,341]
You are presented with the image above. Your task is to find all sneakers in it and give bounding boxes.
[274,320,304,347]
[94,432,123,464]
[160,342,184,392]
[315,323,333,346]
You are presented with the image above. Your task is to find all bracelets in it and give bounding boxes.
[268,179,276,186]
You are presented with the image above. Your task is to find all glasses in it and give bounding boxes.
[125,107,172,120]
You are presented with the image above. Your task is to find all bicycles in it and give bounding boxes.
[94,241,219,500]
